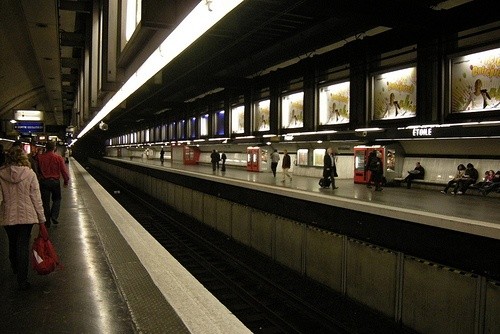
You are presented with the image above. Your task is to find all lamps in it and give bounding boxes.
[317,68,327,84]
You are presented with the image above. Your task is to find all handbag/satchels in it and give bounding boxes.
[30,233,64,274]
[319,176,330,187]
[28,264,56,289]
[368,159,380,173]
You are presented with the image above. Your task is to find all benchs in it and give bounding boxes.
[197,162,247,168]
[394,178,473,193]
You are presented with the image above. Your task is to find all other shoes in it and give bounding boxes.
[51,216,58,224]
[457,191,462,194]
[451,192,455,194]
[46,217,50,223]
[441,190,446,194]
[21,283,30,290]
[333,187,339,189]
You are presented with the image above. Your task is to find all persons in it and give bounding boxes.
[381,93,401,118]
[215,150,220,169]
[460,79,493,109]
[288,109,298,126]
[211,149,217,171]
[270,149,280,178]
[260,114,266,128]
[403,162,425,189]
[322,147,339,190]
[160,148,165,159]
[327,102,340,122]
[368,150,384,191]
[0,140,45,177]
[470,169,500,196]
[221,152,227,171]
[440,164,466,194]
[35,142,69,227]
[282,149,292,182]
[0,147,46,288]
[457,162,478,194]
